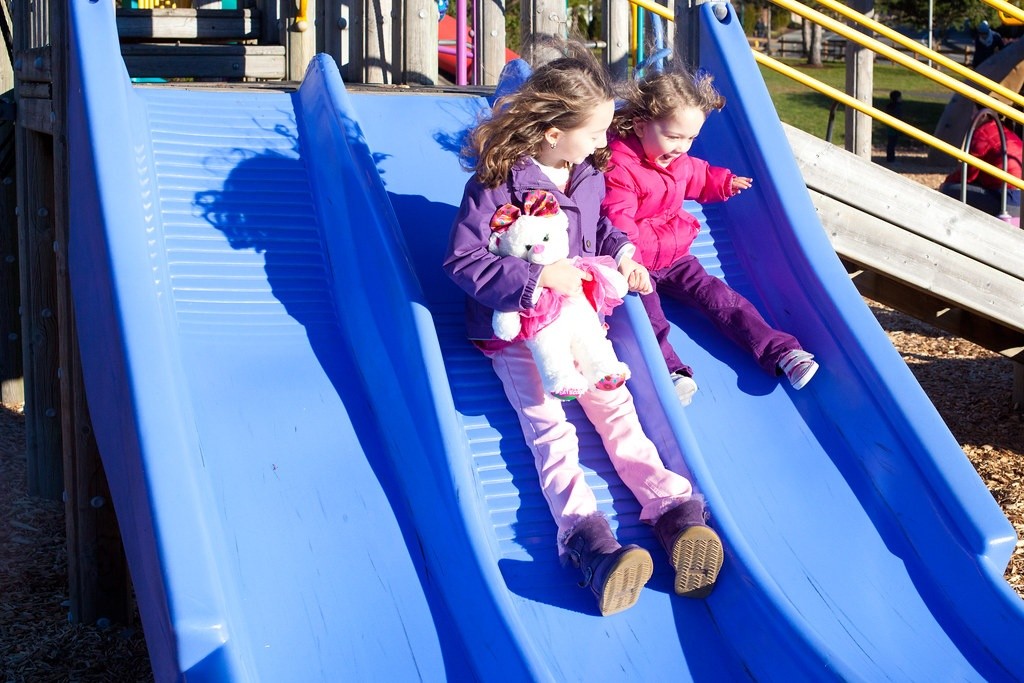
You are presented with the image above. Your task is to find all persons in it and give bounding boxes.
[885,91,903,162]
[441,22,724,616]
[942,98,1024,216]
[973,20,1004,110]
[599,71,819,406]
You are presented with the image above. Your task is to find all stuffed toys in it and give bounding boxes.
[490,190,631,401]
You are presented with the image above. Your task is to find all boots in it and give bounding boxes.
[654,493,723,599]
[559,512,653,617]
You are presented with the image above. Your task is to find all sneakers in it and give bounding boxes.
[779,350,818,390]
[671,374,697,406]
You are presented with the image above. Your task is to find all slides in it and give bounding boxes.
[63,0,1024,683]
